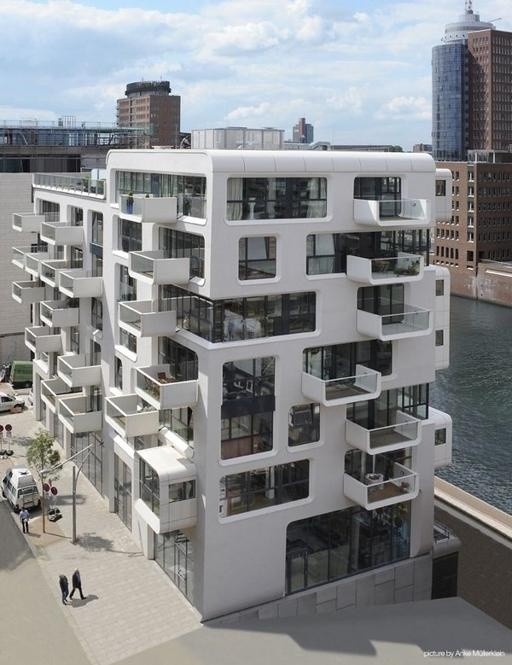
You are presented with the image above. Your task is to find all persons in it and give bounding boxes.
[58,572,70,605]
[69,568,87,600]
[19,505,31,534]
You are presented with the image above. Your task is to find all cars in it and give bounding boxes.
[0,393,25,413]
[0,363,11,383]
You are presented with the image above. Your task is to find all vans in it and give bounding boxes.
[9,360,33,388]
[1,466,41,515]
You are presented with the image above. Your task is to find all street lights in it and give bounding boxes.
[73,442,104,542]
